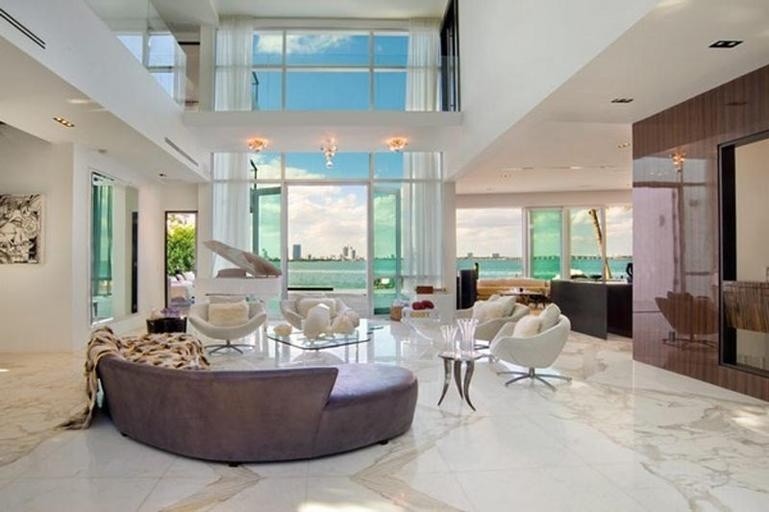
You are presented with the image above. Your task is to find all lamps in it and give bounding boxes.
[319,142,337,168]
[388,137,409,151]
[670,151,687,172]
[246,138,268,152]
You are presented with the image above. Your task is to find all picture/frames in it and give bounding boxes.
[0,190,44,265]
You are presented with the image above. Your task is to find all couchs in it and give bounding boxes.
[477,278,550,301]
[95,325,417,466]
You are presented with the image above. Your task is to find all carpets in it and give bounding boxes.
[0,352,97,469]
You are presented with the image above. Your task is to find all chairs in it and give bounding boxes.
[489,314,573,393]
[189,300,266,355]
[454,300,530,363]
[656,298,719,353]
[281,298,359,331]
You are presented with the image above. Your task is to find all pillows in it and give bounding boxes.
[209,294,246,305]
[488,295,517,316]
[513,314,543,339]
[667,291,692,300]
[472,300,504,324]
[298,299,338,319]
[209,300,248,326]
[538,303,561,332]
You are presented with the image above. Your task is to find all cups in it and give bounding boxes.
[455,318,480,355]
[440,324,459,354]
